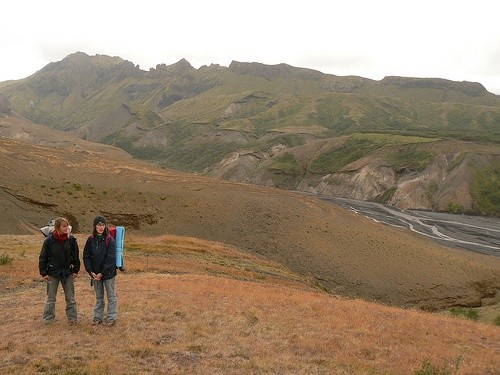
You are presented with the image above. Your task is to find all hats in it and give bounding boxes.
[93,216,107,226]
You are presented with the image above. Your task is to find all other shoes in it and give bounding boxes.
[92,317,102,325]
[104,318,117,325]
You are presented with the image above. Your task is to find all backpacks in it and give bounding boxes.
[104,226,127,272]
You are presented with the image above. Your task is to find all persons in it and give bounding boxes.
[38,218,81,325]
[83,216,118,325]
